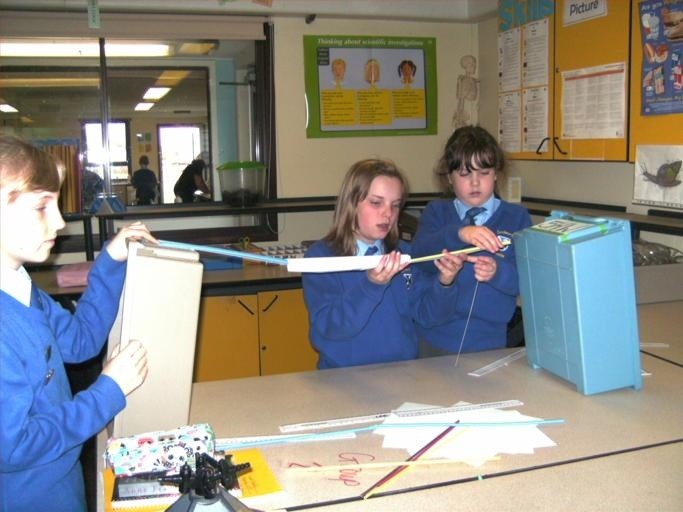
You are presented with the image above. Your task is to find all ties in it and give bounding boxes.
[463,208,485,225]
[366,246,378,256]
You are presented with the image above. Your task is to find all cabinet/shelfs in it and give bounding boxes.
[192,261,319,385]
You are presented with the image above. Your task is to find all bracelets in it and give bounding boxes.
[438,278,456,287]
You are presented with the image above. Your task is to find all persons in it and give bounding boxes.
[407,125,536,359]
[398,59,417,82]
[174,151,210,204]
[332,57,345,85]
[131,154,157,205]
[0,136,150,512]
[302,159,467,369]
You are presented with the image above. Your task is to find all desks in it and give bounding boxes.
[28,347,683,512]
